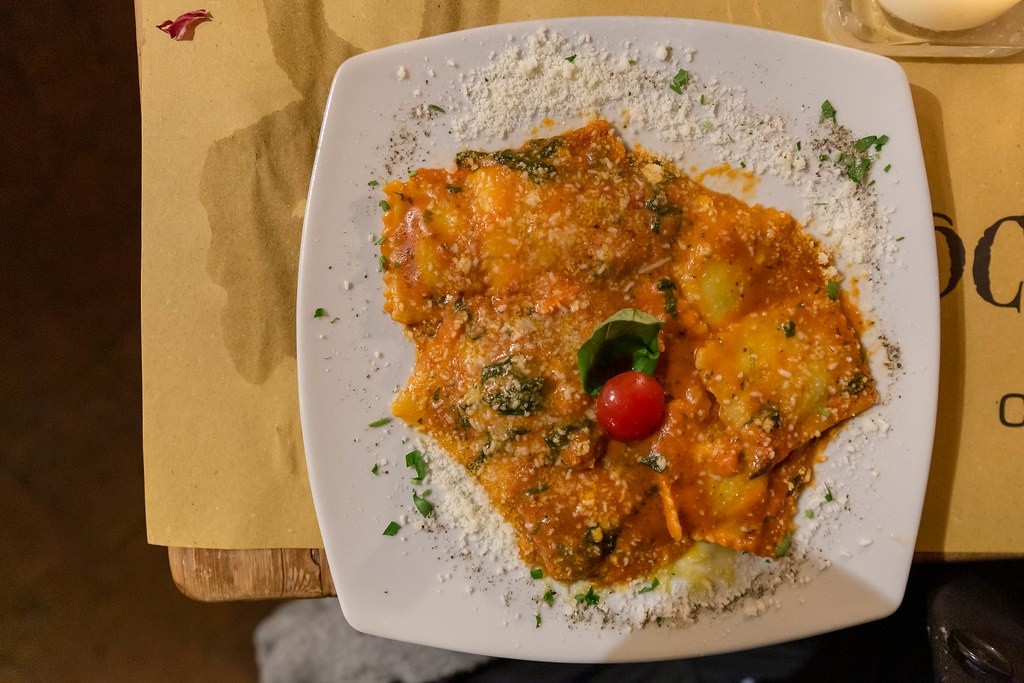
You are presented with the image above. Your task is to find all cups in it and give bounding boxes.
[828,0,1024,57]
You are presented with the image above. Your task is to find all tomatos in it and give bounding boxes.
[595,370,667,441]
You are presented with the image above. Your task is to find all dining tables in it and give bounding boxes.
[133,0,1024,664]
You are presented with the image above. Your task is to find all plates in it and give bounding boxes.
[299,16,940,664]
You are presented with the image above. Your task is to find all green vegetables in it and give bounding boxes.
[312,67,891,627]
